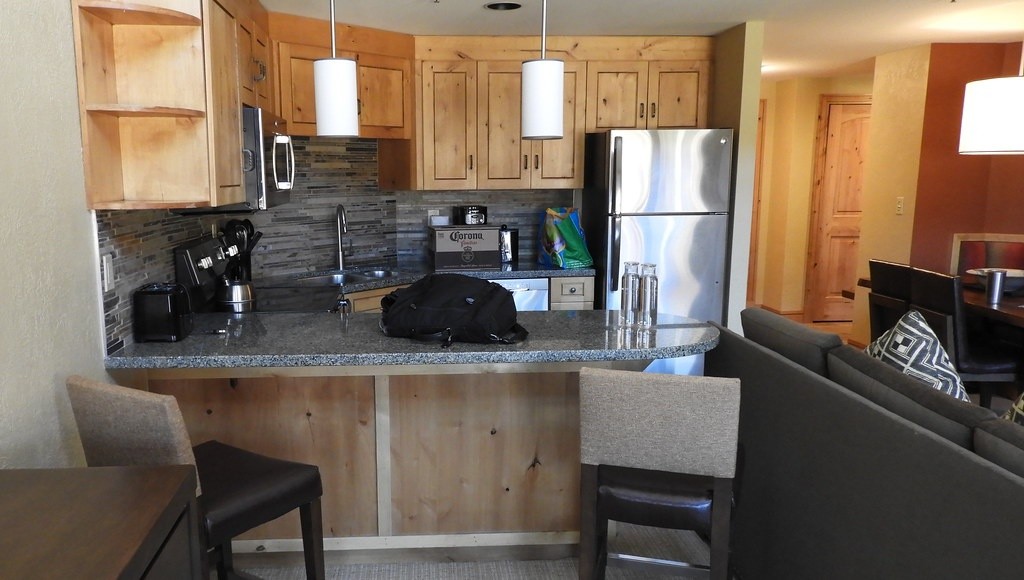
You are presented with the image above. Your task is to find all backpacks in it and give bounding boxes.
[378,271,529,348]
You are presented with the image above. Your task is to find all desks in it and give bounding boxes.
[857,273,1024,401]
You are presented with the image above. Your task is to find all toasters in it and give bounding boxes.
[133,282,193,342]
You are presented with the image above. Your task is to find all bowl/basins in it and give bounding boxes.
[965,268,1024,291]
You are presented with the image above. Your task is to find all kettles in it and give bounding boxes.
[218,261,253,302]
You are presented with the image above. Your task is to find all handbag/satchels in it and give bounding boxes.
[538,208,594,268]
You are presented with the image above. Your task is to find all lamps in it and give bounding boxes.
[312,0,359,139]
[958,76,1024,155]
[520,0,565,140]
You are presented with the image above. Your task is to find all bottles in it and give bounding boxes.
[620,262,658,326]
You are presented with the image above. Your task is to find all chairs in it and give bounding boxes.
[869,233,1024,409]
[66,374,326,580]
[579,367,741,580]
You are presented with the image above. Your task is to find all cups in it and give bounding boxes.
[986,270,1007,304]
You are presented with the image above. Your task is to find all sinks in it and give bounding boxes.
[359,269,398,278]
[297,273,363,285]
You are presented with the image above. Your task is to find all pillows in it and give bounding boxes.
[861,308,970,406]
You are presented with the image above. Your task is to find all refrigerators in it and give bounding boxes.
[581,128,733,377]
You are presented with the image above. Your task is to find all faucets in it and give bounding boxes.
[336,203,347,270]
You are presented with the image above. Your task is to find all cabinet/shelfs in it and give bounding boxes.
[71,0,715,213]
[341,283,413,313]
[0,467,204,580]
[548,277,595,311]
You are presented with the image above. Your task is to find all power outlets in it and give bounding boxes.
[427,210,439,226]
[895,196,903,215]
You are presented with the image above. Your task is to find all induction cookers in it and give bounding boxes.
[173,232,350,313]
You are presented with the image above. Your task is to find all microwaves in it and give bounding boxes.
[169,107,295,215]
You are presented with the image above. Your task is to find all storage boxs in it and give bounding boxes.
[429,225,501,272]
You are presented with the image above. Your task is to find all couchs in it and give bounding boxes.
[701,305,1024,580]
